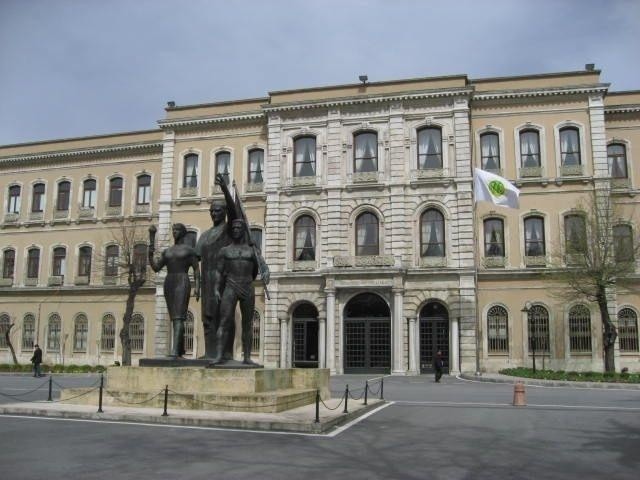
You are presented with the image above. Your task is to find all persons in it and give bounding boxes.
[30,345,42,377]
[148,173,270,366]
[434,350,443,383]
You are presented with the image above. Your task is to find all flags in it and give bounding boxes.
[475,168,521,209]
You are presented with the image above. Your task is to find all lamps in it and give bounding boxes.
[585,63,596,71]
[167,99,175,107]
[360,75,369,85]
